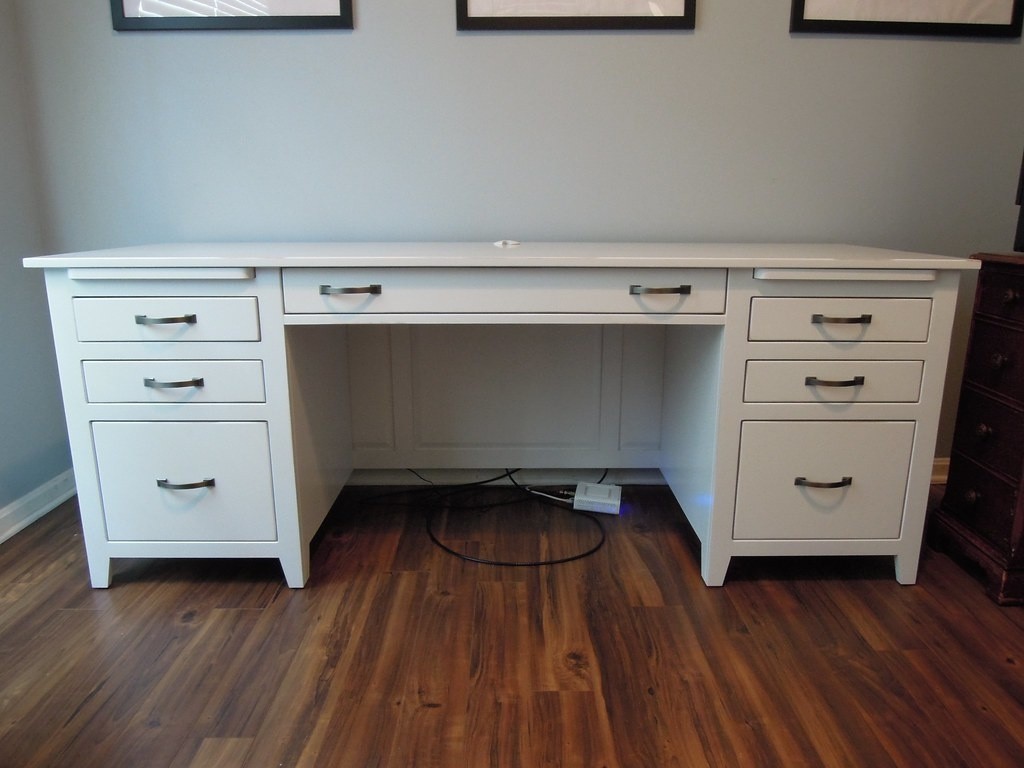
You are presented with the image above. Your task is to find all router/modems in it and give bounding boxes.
[573,482,622,514]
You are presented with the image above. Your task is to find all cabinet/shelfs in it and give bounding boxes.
[927,253,1024,605]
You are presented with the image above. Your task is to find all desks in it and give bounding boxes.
[23,245,983,588]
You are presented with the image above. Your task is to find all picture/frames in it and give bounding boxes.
[789,0,1024,38]
[456,0,695,30]
[110,1,353,31]
